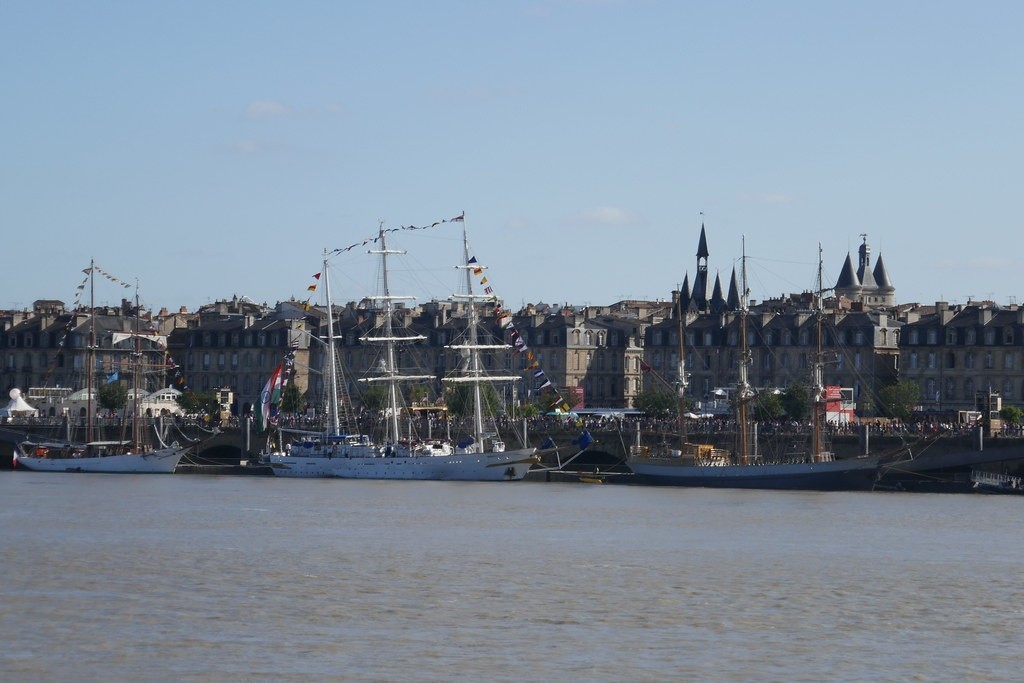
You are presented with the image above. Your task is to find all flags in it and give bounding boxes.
[261,360,283,431]
[13,451,18,466]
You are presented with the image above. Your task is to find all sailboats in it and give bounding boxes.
[241,209,600,481]
[15,259,203,474]
[620,231,947,489]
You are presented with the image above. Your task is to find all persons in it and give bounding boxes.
[96,407,1024,456]
